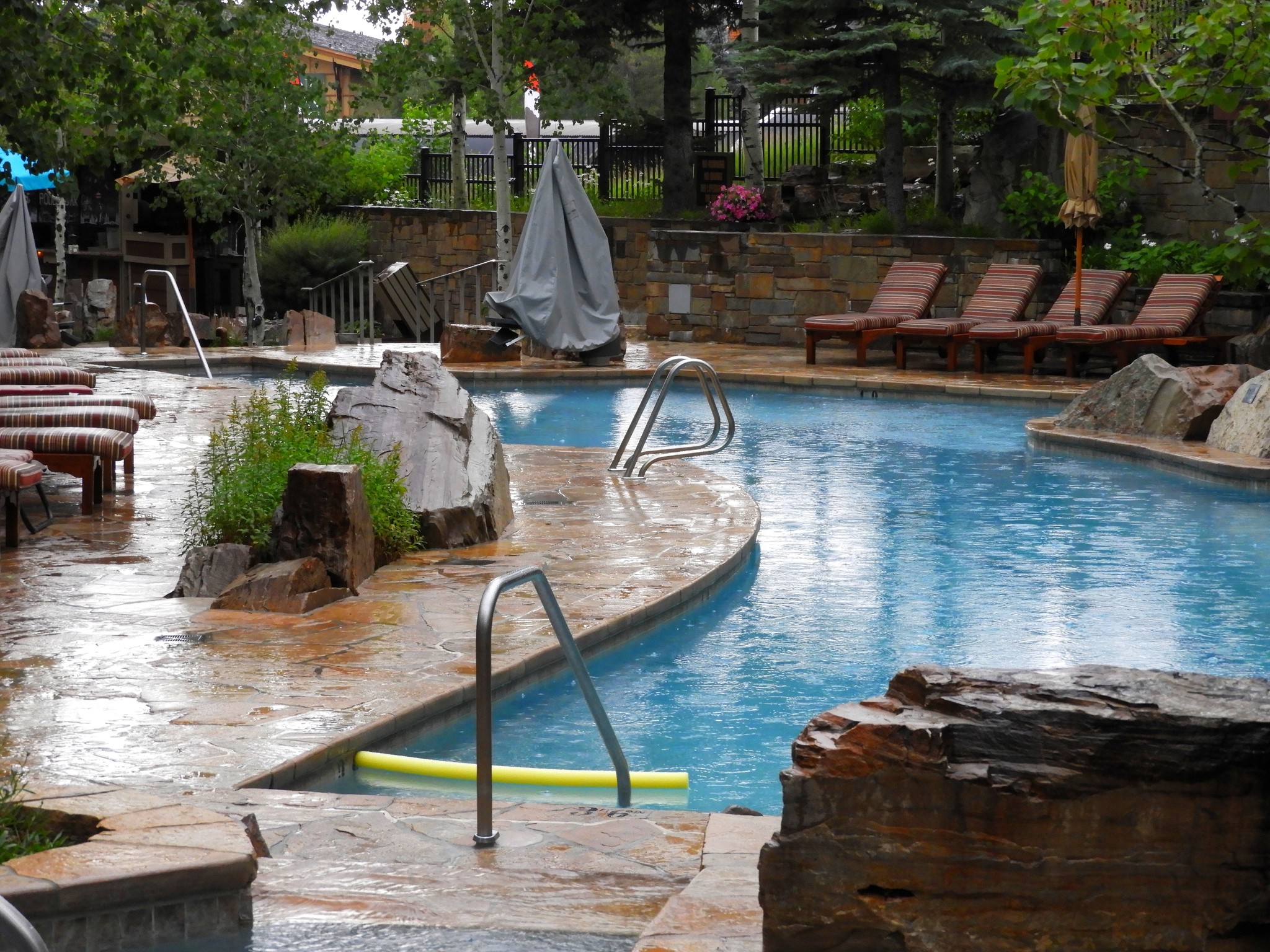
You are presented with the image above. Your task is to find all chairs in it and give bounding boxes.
[805,263,947,368]
[0,347,157,548]
[969,269,1134,372]
[896,265,1043,371]
[1056,274,1225,377]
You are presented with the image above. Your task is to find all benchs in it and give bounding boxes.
[1163,332,1243,365]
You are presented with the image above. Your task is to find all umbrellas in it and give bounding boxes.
[1058,97,1103,326]
[0,148,72,195]
[115,148,246,258]
